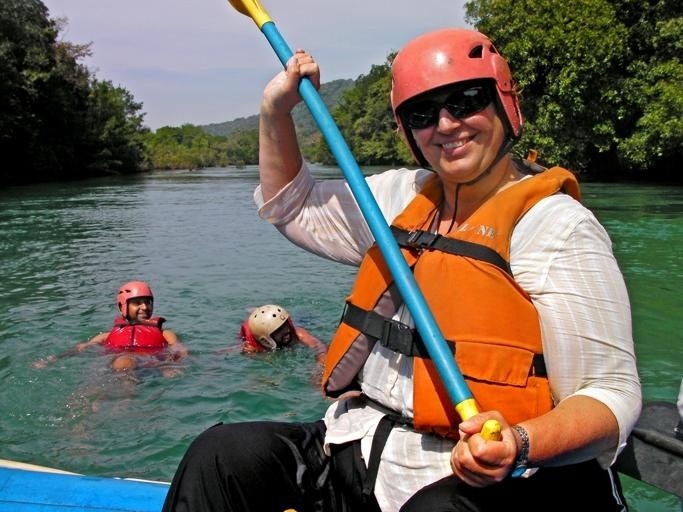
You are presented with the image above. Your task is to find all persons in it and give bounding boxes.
[29,281,186,379]
[161,28,644,512]
[216,304,328,389]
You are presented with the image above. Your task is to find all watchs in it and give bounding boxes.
[510,423,530,479]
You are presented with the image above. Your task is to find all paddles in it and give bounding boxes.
[611,400,683,498]
[229,0,501,442]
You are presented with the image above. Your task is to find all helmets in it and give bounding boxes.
[387,25,523,169]
[246,303,296,353]
[114,278,155,322]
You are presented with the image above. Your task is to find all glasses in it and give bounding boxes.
[402,83,495,131]
